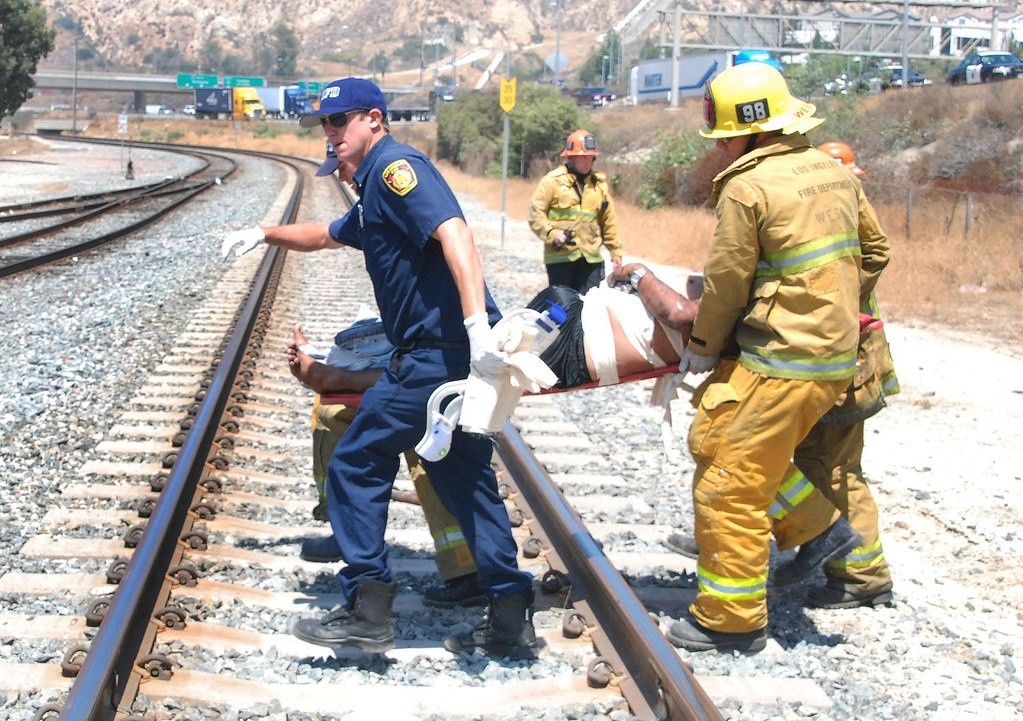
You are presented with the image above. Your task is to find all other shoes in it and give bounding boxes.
[313,505,329,521]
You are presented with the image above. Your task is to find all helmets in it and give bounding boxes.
[559,130,600,156]
[818,141,865,177]
[699,62,827,138]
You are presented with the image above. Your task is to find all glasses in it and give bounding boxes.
[320,109,365,128]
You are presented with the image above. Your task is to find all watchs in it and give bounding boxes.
[631,268,653,291]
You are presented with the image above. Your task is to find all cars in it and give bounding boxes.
[184,105,196,115]
[571,89,617,108]
[825,49,1023,97]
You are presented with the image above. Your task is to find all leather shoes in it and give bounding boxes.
[773,515,863,586]
[807,587,893,607]
[666,619,766,651]
[421,572,490,605]
[299,534,345,563]
[663,533,702,559]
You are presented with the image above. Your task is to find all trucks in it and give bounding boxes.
[256,84,314,120]
[146,105,173,116]
[382,88,457,121]
[194,87,267,122]
[637,50,783,97]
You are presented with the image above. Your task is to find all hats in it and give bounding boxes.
[315,137,342,176]
[299,78,388,128]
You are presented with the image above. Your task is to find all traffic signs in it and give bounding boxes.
[223,77,264,87]
[176,73,218,90]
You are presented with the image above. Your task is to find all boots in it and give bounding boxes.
[292,579,401,653]
[444,587,541,659]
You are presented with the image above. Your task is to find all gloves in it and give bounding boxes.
[678,344,722,375]
[464,311,510,375]
[221,224,266,263]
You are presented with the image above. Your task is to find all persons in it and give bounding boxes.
[664,63,863,650]
[309,137,487,606]
[665,142,899,611]
[286,263,704,394]
[528,130,622,295]
[221,78,534,663]
